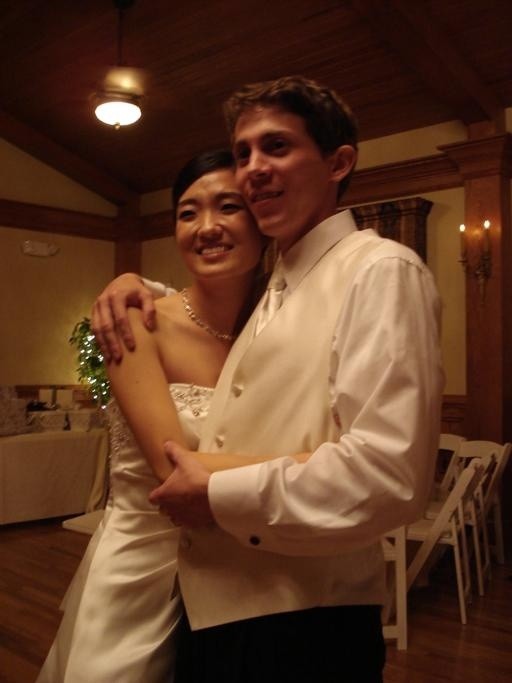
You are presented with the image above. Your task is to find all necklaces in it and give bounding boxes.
[179,286,237,345]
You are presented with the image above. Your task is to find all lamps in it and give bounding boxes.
[86,2,145,130]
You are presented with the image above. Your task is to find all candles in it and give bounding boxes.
[459,224,466,257]
[482,219,490,253]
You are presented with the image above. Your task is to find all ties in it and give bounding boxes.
[252,263,286,336]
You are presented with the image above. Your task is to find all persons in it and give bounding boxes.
[88,75,444,683]
[34,150,274,683]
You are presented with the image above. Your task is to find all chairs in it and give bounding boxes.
[380,433,512,651]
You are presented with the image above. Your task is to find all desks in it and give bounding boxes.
[1,427,109,527]
